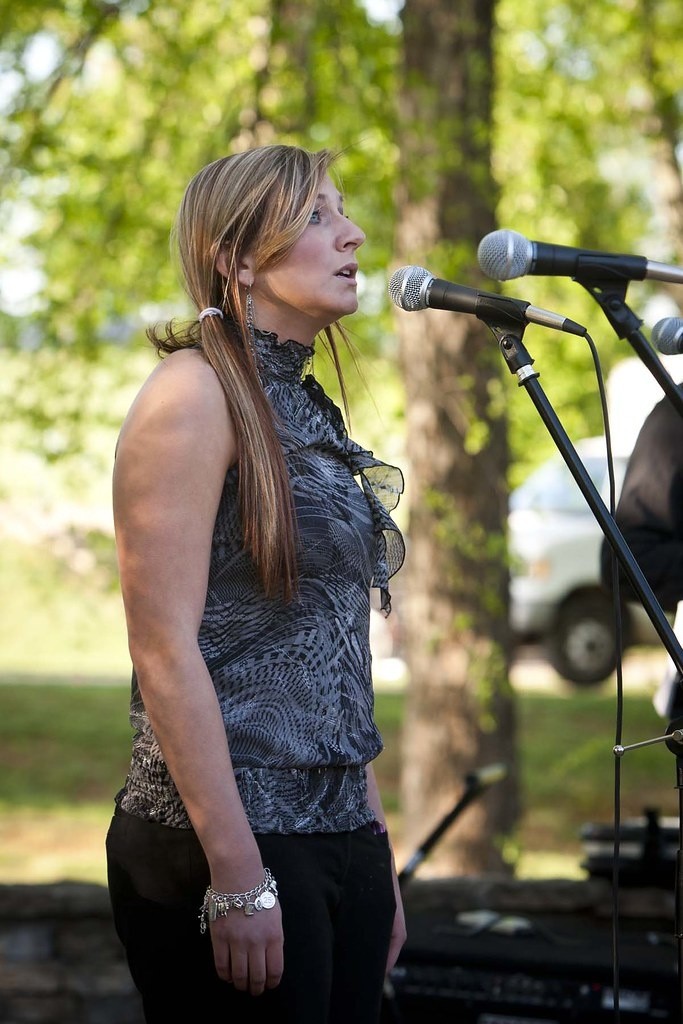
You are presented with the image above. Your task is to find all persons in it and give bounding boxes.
[598,374,683,754]
[104,145,407,1024]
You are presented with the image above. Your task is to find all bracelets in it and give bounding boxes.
[199,865,279,934]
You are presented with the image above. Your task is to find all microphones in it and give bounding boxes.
[387,266,587,338]
[477,229,683,285]
[651,317,683,355]
